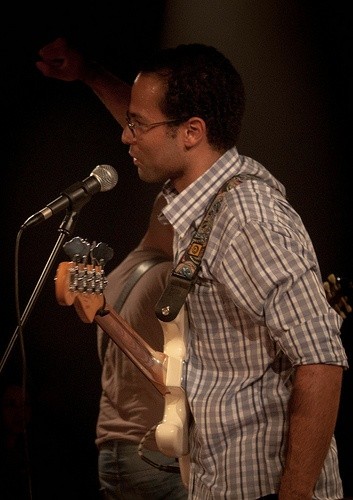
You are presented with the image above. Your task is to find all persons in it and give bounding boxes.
[95,188,191,500]
[0,383,49,500]
[35,37,349,500]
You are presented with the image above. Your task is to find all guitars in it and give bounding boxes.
[53,235,192,486]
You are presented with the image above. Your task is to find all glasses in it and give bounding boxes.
[124,114,188,133]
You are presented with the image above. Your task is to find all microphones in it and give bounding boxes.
[22,165,118,230]
